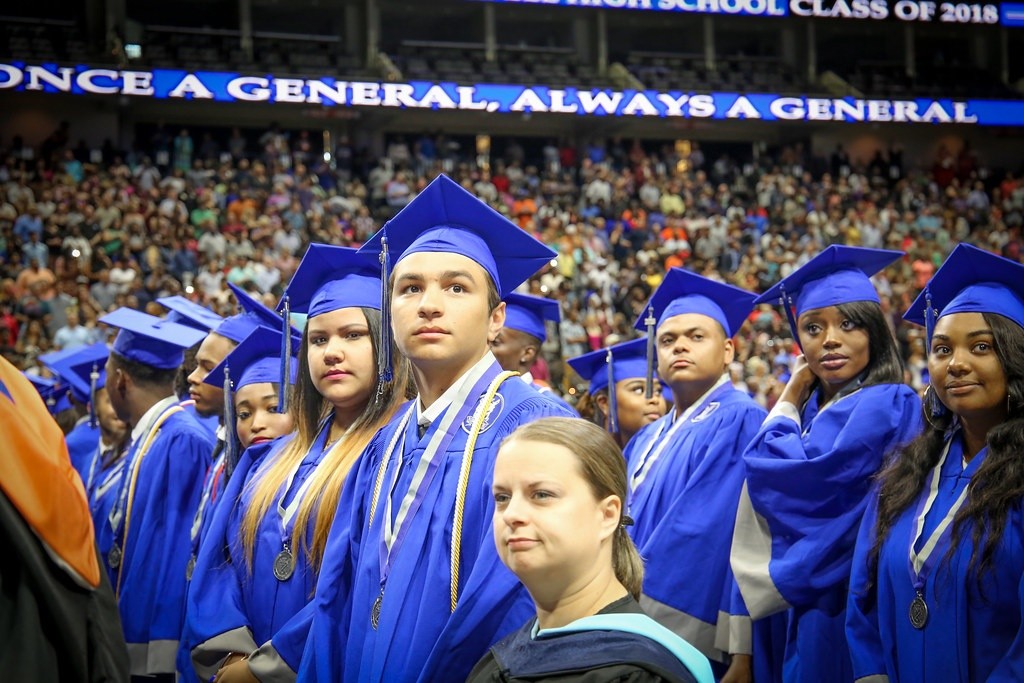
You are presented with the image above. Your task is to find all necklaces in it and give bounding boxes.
[324,435,339,451]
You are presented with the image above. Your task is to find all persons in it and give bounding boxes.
[186,278,304,607]
[465,414,716,683]
[0,286,223,683]
[567,334,668,452]
[615,264,788,683]
[201,324,305,458]
[182,240,390,683]
[0,139,1024,371]
[500,284,580,417]
[296,174,559,683]
[729,242,927,683]
[844,243,1024,683]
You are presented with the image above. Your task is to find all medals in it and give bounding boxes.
[273,552,295,581]
[622,507,633,530]
[186,559,194,581]
[108,543,122,568]
[371,595,384,630]
[907,591,928,629]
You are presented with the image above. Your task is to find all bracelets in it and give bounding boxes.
[218,650,235,670]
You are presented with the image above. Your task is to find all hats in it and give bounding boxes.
[25,172,558,487]
[633,267,760,398]
[902,243,1024,417]
[752,244,905,354]
[566,337,658,433]
[501,291,561,343]
